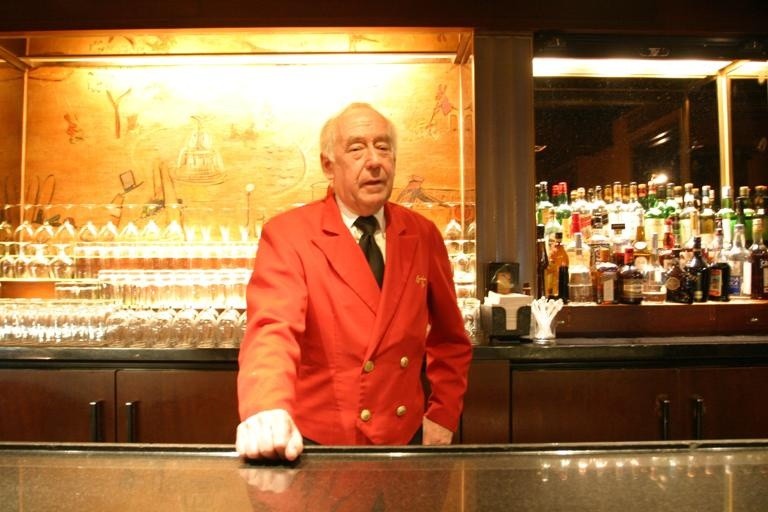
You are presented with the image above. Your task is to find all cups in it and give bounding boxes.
[182,209,195,242]
[191,246,208,269]
[189,269,218,349]
[255,208,265,239]
[54,204,77,242]
[123,269,155,349]
[98,204,119,242]
[163,204,184,242]
[237,246,249,268]
[27,244,49,279]
[77,204,98,243]
[49,244,74,279]
[200,209,213,241]
[105,248,118,272]
[131,247,144,270]
[0,205,13,241]
[443,202,462,240]
[145,248,160,269]
[208,246,222,269]
[74,247,90,279]
[89,246,105,279]
[141,203,162,240]
[239,208,250,242]
[237,269,251,345]
[106,269,127,348]
[119,247,130,270]
[120,204,140,242]
[161,248,176,270]
[36,205,55,243]
[13,204,36,242]
[219,208,233,242]
[466,203,476,240]
[145,270,176,349]
[177,247,191,269]
[54,282,101,299]
[13,242,30,279]
[0,242,15,280]
[0,298,115,343]
[168,270,197,349]
[223,246,236,269]
[450,241,473,282]
[212,270,237,348]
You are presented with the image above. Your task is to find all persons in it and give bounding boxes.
[235,102,472,461]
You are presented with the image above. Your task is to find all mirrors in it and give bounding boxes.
[533,31,768,285]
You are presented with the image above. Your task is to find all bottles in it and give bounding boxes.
[638,184,647,203]
[603,185,613,204]
[715,187,737,252]
[739,186,755,245]
[536,181,553,225]
[666,245,694,303]
[708,228,730,301]
[627,182,644,241]
[748,219,768,298]
[754,186,768,245]
[536,224,549,298]
[729,224,752,299]
[555,182,572,233]
[644,233,667,302]
[570,234,593,302]
[691,189,700,211]
[597,248,614,302]
[552,185,558,208]
[674,186,684,209]
[617,248,644,303]
[591,184,610,238]
[664,183,682,247]
[623,185,629,204]
[550,233,569,303]
[570,191,577,209]
[659,220,675,267]
[679,185,700,248]
[687,237,710,302]
[684,210,709,262]
[609,182,628,242]
[736,197,747,249]
[644,181,664,248]
[698,186,716,237]
[574,187,589,216]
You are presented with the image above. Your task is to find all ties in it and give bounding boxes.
[354,215,385,290]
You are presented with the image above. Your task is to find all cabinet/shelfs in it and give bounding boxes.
[510,342,768,444]
[0,345,239,442]
